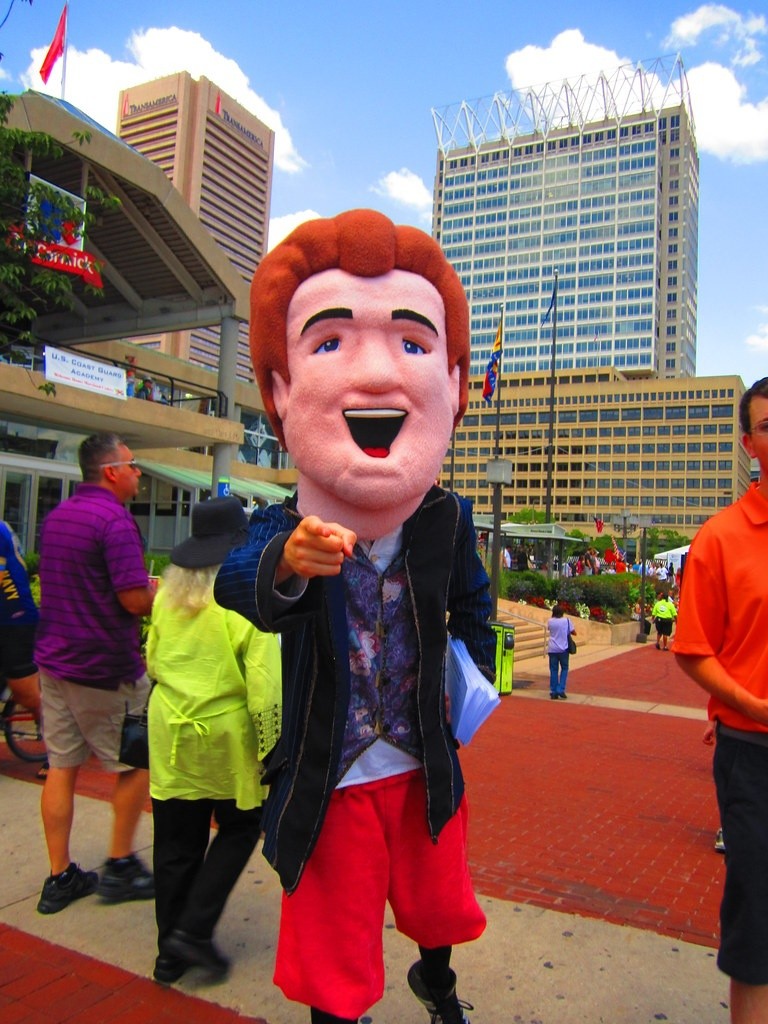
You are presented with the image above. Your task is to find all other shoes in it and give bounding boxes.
[656,644,660,649]
[153,927,229,982]
[551,693,566,699]
[714,829,726,850]
[664,646,668,650]
[35,762,49,778]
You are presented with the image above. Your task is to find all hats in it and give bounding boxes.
[169,497,251,570]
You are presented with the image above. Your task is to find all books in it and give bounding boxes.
[446,627,502,748]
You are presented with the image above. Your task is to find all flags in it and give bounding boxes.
[594,518,604,533]
[542,282,555,327]
[482,321,501,403]
[39,5,66,85]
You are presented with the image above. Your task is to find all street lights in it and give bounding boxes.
[484,456,513,620]
[611,508,640,569]
[636,514,653,643]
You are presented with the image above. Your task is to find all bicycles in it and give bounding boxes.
[0,686,51,764]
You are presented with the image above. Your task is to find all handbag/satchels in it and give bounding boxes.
[567,618,577,655]
[117,682,158,770]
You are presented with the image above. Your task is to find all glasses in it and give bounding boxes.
[99,455,137,470]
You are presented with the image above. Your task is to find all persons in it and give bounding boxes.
[125,352,169,405]
[547,605,577,699]
[502,544,678,651]
[213,205,497,1024]
[671,378,768,1024]
[143,495,282,981]
[702,722,725,852]
[0,519,49,779]
[32,431,156,915]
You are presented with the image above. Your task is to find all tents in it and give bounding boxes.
[654,544,691,574]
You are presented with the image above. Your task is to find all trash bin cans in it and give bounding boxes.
[487,621,516,696]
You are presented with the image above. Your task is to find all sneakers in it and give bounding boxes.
[96,852,154,899]
[37,862,99,915]
[407,959,474,1024]
